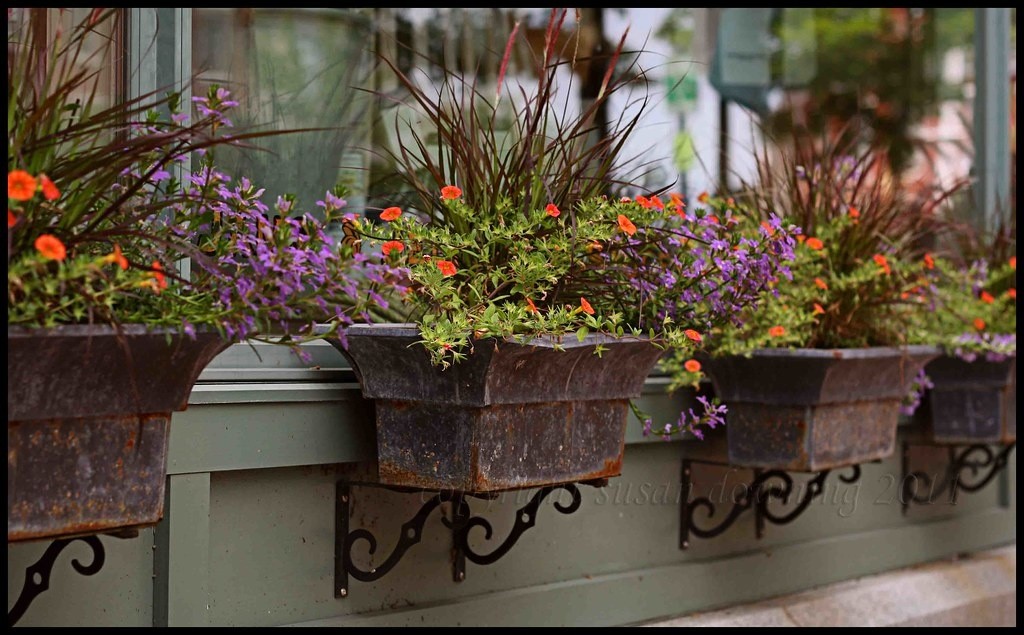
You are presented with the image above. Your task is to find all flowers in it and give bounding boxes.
[698,96,973,413]
[8,8,426,369]
[366,10,800,440]
[928,184,1017,361]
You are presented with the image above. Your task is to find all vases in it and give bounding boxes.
[684,349,941,475]
[343,324,665,496]
[931,355,1017,443]
[7,324,238,541]
